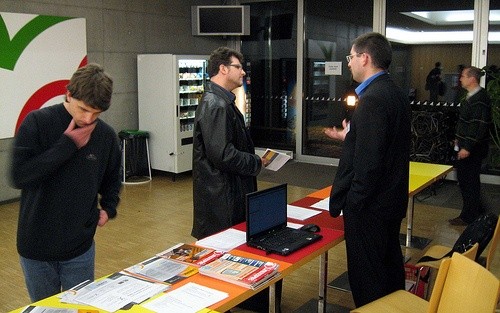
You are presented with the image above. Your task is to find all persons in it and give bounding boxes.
[425,62,443,103]
[8,64,122,303]
[448,67,492,227]
[324,32,409,308]
[191,47,267,240]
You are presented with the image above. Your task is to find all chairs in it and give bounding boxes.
[414,214,500,303]
[350,242,478,313]
[437,251,500,313]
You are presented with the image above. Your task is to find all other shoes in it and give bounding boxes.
[448,217,468,225]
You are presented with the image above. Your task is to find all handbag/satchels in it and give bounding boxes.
[450,212,496,260]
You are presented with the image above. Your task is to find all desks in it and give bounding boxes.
[9,162,454,313]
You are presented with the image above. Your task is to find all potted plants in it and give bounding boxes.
[455,66,500,170]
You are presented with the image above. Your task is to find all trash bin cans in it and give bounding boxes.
[118,127,152,184]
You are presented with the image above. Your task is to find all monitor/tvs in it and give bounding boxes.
[191,4,251,36]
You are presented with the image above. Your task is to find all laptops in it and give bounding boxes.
[244,183,322,255]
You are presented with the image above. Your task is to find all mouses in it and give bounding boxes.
[300,224,320,233]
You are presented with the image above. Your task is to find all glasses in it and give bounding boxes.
[346,51,368,62]
[225,65,243,70]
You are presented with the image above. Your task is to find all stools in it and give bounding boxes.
[118,129,152,185]
